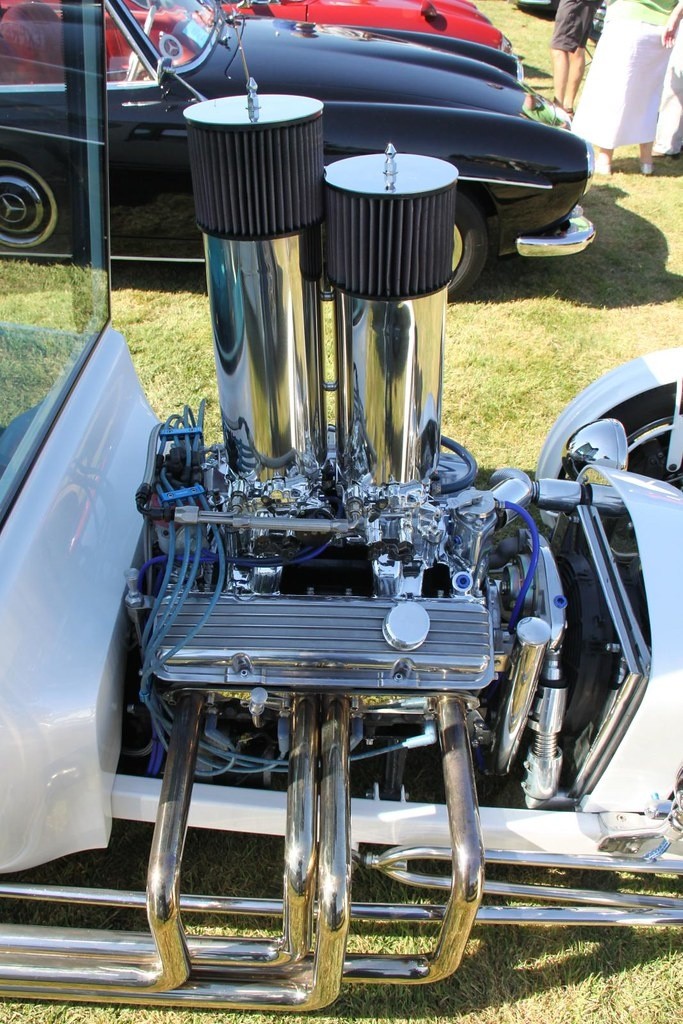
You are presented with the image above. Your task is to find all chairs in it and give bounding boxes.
[1,2,62,85]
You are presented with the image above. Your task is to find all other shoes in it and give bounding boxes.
[640,158,655,175]
[596,159,613,177]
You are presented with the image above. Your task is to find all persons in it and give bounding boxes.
[549,1,683,176]
[521,82,570,131]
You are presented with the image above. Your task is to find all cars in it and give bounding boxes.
[0,1,597,295]
